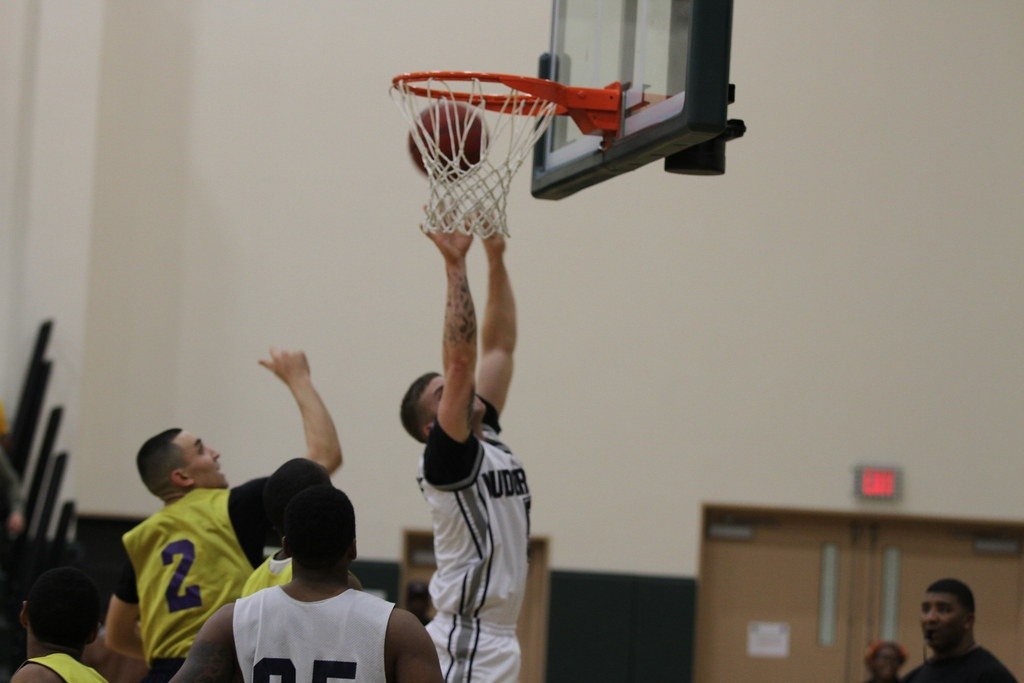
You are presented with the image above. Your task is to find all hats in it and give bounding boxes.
[865,642,907,667]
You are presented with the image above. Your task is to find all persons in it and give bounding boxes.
[865,579,1016,682]
[104,347,343,683]
[400,200,531,682]
[11,567,107,682]
[168,456,444,683]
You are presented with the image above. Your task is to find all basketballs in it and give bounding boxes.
[406,100,490,185]
